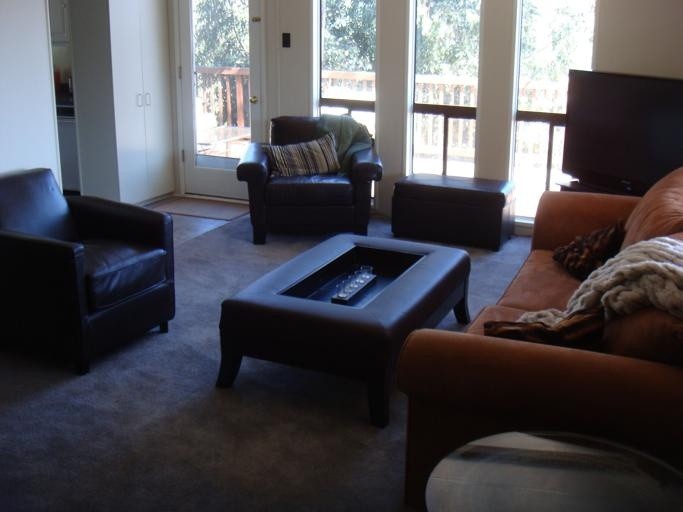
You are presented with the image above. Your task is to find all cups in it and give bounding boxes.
[336,265,373,298]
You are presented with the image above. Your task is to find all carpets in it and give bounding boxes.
[144,196,249,222]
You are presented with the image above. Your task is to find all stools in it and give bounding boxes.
[423,431,682,511]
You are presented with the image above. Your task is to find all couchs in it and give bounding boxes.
[397,166,683,510]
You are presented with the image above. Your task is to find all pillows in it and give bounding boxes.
[259,135,341,180]
[480,305,608,350]
[550,216,626,277]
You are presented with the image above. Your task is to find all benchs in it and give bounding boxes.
[390,171,517,255]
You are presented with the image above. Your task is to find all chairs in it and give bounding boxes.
[236,111,381,243]
[0,166,176,376]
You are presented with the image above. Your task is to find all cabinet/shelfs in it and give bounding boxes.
[66,1,178,208]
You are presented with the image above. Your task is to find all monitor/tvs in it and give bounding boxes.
[560,67,683,197]
